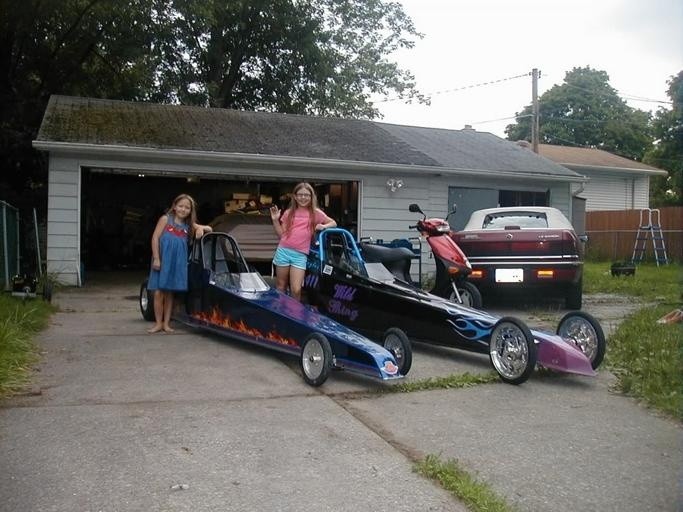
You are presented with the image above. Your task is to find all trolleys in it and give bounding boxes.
[359,235,422,290]
[0,273,52,304]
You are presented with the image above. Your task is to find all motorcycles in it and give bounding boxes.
[355,204,483,313]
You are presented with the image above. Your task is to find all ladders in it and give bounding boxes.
[631,208,669,268]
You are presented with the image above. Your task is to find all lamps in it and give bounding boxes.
[386,178,404,193]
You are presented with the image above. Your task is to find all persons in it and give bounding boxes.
[269,182,337,302]
[146,194,212,333]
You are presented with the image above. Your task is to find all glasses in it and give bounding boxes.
[295,193,311,198]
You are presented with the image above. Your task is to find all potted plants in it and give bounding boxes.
[610,256,636,277]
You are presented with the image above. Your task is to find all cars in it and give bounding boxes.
[205,214,280,262]
[450,204,590,310]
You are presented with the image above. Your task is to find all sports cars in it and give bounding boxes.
[286,228,608,384]
[138,231,413,387]
